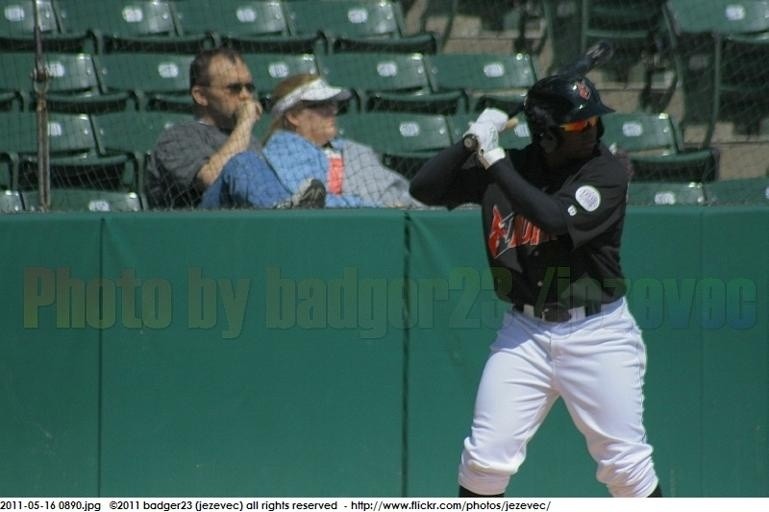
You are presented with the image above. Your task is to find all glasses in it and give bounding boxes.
[227,82,256,94]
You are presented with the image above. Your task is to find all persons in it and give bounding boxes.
[259,71,433,209]
[405,71,669,496]
[142,49,326,214]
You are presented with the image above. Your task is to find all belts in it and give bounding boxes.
[514,300,601,322]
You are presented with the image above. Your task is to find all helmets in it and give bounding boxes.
[523,73,616,144]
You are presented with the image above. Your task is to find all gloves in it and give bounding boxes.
[462,108,508,170]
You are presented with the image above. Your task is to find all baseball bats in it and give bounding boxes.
[461,39,617,151]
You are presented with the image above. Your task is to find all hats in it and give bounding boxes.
[270,78,351,120]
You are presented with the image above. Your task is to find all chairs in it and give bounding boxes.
[1,1,768,215]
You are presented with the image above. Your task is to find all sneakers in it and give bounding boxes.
[272,177,326,209]
